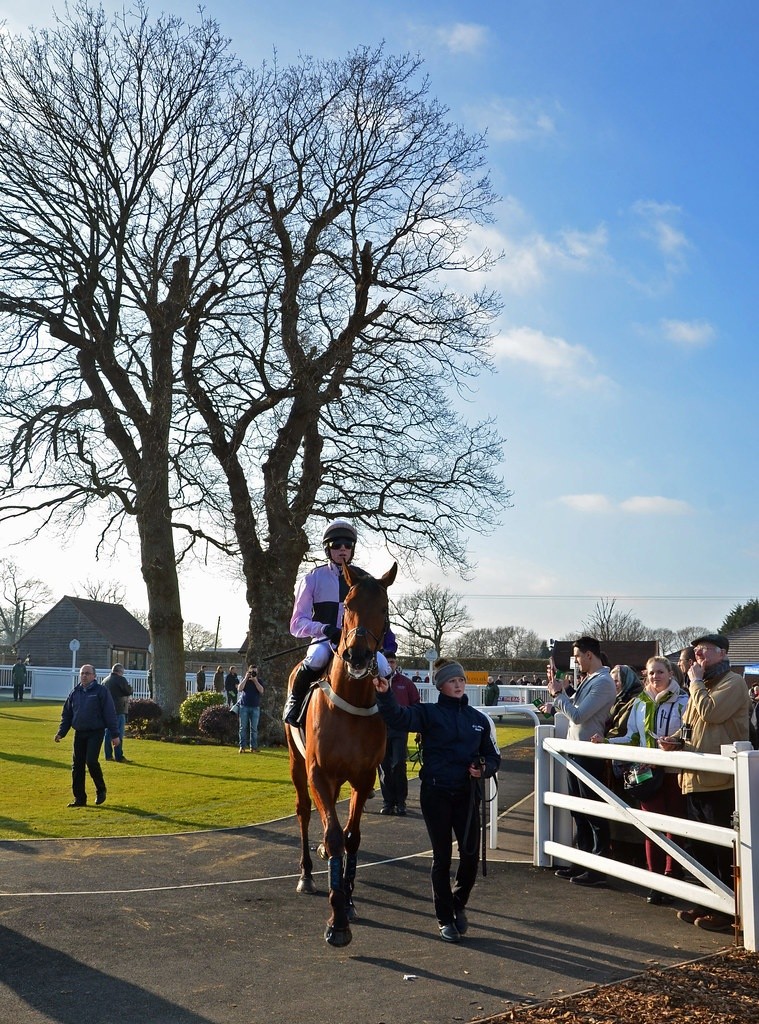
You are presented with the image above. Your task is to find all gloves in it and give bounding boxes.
[324,625,342,645]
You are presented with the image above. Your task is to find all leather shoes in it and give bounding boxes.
[67,799,87,807]
[436,908,469,943]
[94,787,107,804]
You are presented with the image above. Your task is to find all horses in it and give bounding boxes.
[285,556,398,947]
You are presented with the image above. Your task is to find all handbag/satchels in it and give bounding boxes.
[230,702,240,715]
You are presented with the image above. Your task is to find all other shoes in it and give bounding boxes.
[106,756,133,762]
[555,855,608,886]
[238,747,260,753]
[677,908,734,931]
[498,715,503,722]
[380,801,406,815]
[647,875,680,904]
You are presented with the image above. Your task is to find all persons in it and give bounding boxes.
[197,665,206,692]
[55,664,120,807]
[484,676,503,721]
[225,666,240,707]
[377,652,420,814]
[101,663,133,761]
[373,658,501,942]
[238,665,265,754]
[213,665,224,692]
[11,653,32,701]
[412,671,435,702]
[495,634,759,928]
[148,663,153,699]
[282,521,377,728]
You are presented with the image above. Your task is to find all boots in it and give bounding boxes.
[283,663,320,728]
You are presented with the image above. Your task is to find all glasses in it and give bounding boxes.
[79,672,94,676]
[329,538,355,549]
[693,646,717,652]
[677,658,689,662]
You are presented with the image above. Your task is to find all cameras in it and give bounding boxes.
[229,699,240,715]
[249,670,257,677]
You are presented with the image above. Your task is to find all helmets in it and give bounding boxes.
[322,522,357,559]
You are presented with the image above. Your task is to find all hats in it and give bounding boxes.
[691,634,729,654]
